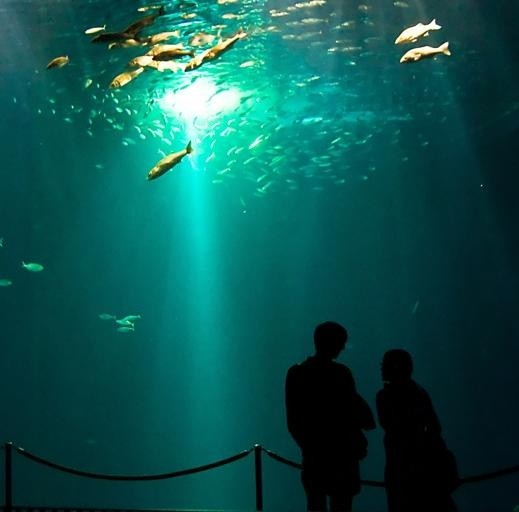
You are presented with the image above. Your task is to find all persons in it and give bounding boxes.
[285,322,376,512]
[376,348,460,512]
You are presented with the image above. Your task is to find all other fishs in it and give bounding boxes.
[11,0,476,198]
